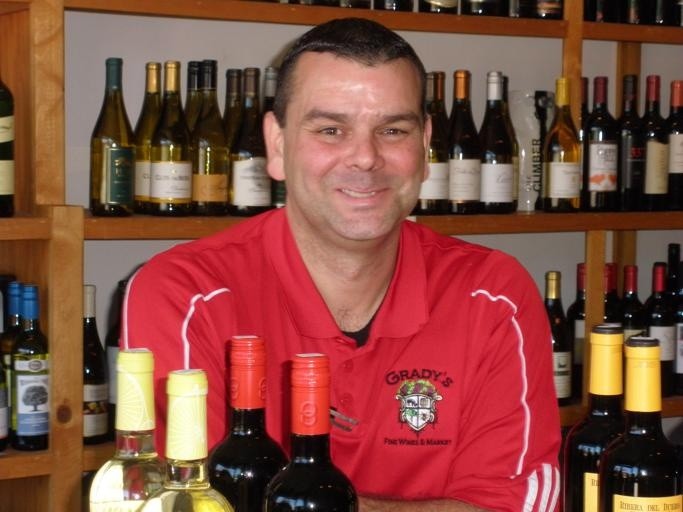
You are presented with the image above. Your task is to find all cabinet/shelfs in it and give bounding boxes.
[0,0,683,512]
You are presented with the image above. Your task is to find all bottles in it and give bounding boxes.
[0,270,48,453]
[263,351,361,512]
[562,326,682,510]
[87,347,169,512]
[89,58,285,215]
[540,244,682,406]
[0,74,15,216]
[338,0,682,28]
[138,369,235,512]
[206,335,290,511]
[418,70,682,213]
[79,284,109,439]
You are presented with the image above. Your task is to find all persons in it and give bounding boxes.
[121,18,562,511]
